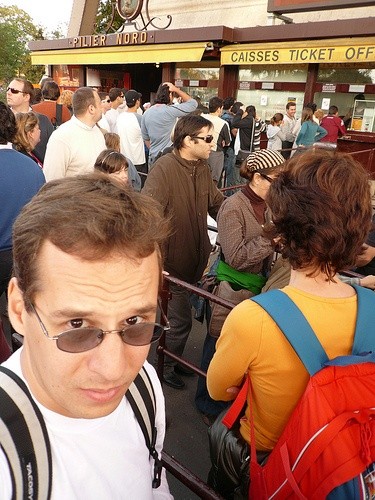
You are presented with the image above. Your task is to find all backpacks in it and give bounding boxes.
[246,283,375,500]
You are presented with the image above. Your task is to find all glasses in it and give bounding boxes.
[193,135,213,144]
[7,87,27,95]
[119,95,124,99]
[102,98,111,104]
[28,297,171,354]
[258,171,274,184]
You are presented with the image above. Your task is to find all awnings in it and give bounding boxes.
[29,43,211,66]
[220,36,375,65]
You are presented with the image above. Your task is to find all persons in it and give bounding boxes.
[343,94,368,128]
[262,209,375,292]
[207,149,375,500]
[141,115,229,390]
[0,168,175,500]
[195,149,288,428]
[5,74,347,210]
[0,101,46,356]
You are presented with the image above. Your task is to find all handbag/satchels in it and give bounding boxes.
[207,279,255,338]
[233,149,252,169]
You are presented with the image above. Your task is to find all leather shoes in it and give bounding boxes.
[164,373,186,389]
[173,363,197,376]
